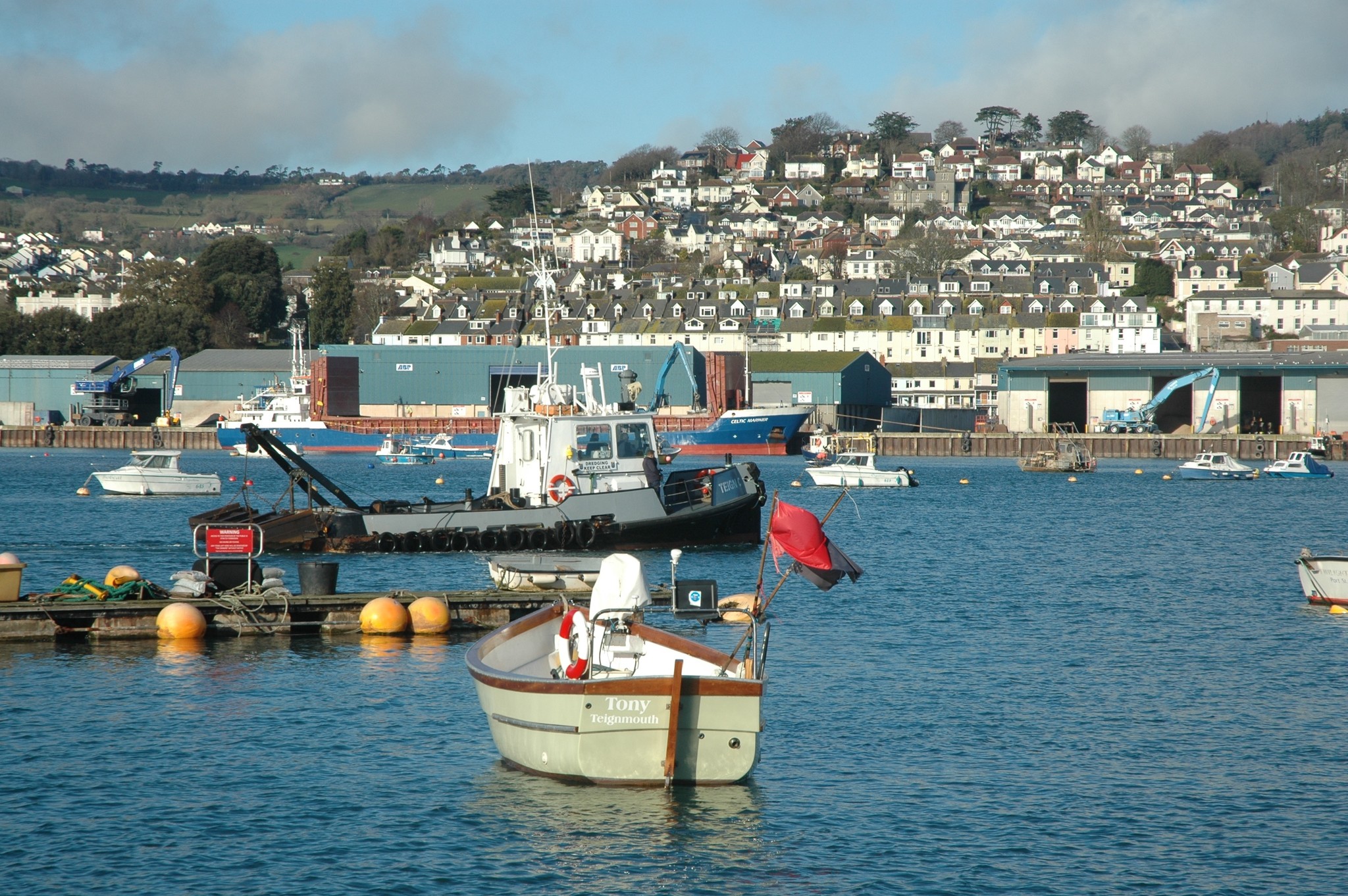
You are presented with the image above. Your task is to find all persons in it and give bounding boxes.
[815,450,828,466]
[1249,416,1275,434]
[815,438,821,447]
[586,433,609,456]
[219,414,224,421]
[642,449,663,500]
[617,433,638,457]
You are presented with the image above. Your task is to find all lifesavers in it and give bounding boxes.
[151,425,162,447]
[44,425,53,445]
[376,519,595,555]
[868,432,878,464]
[694,468,717,493]
[1255,436,1265,459]
[548,474,574,503]
[961,431,971,451]
[557,609,590,680]
[301,380,306,385]
[1341,441,1348,457]
[1152,440,1161,455]
[1322,436,1331,457]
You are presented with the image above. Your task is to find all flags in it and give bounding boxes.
[770,500,864,592]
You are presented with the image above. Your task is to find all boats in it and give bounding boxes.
[371,433,437,467]
[1016,421,1099,472]
[463,553,774,788]
[1300,438,1328,460]
[188,163,768,556]
[641,432,681,463]
[1294,548,1348,606]
[1263,451,1334,479]
[1179,452,1259,480]
[802,426,876,468]
[805,452,920,487]
[214,322,814,455]
[489,555,606,593]
[91,450,221,496]
[401,432,494,460]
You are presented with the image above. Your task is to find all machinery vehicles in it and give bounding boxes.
[636,341,707,415]
[73,346,183,428]
[1101,366,1220,434]
